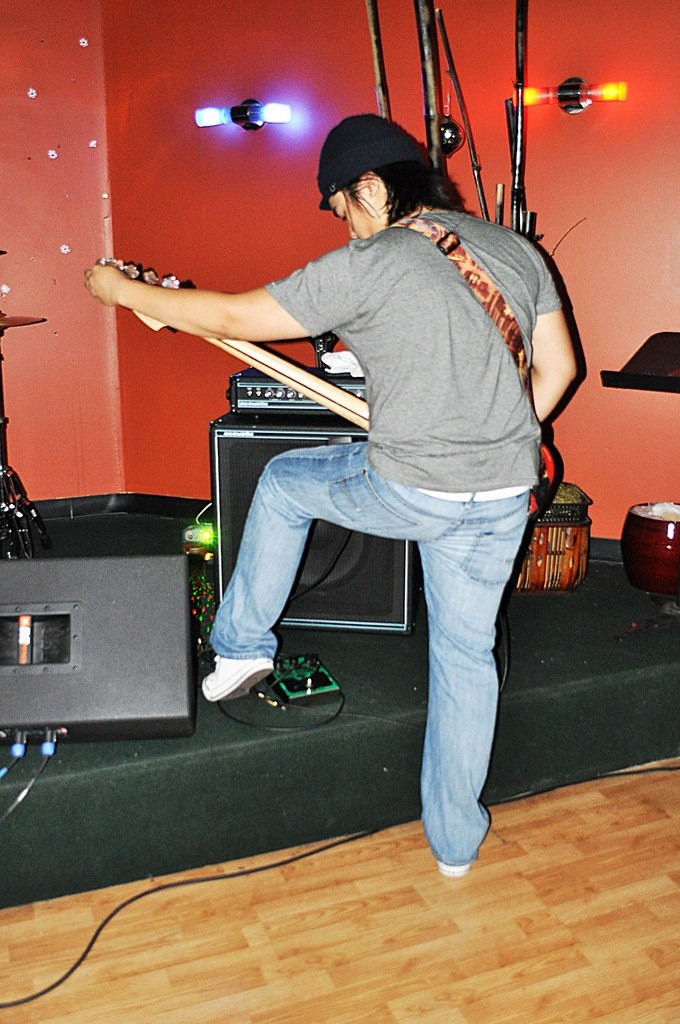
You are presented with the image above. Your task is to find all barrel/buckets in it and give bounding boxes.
[620,502,680,594]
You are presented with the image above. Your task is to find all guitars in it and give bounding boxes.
[92,260,560,519]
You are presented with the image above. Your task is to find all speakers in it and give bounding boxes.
[0,556,200,747]
[209,413,423,636]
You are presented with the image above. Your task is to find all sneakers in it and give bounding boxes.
[437,858,475,878]
[200,655,274,702]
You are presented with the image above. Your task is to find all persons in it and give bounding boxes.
[85,113,577,877]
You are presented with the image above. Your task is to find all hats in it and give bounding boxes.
[317,113,430,210]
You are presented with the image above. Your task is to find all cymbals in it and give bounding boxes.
[1,312,47,328]
